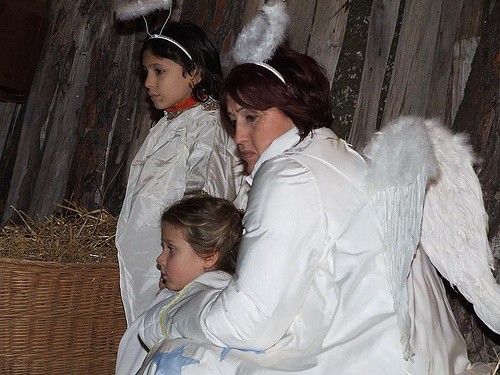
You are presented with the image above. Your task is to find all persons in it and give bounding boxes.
[113,192,245,375]
[116,21,245,325]
[136,49,471,375]
